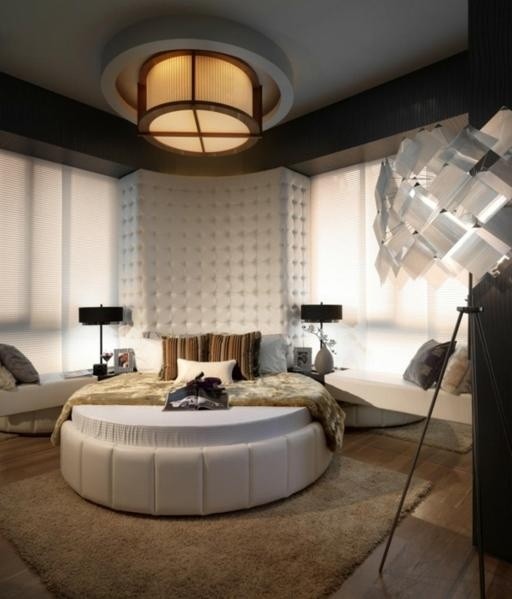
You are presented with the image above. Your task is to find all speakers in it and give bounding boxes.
[301,304,343,324]
[78,307,124,325]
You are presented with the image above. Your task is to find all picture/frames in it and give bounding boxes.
[293,346,313,373]
[113,347,135,374]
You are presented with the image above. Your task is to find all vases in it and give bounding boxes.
[314,344,334,374]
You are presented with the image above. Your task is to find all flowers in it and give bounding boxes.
[301,325,338,354]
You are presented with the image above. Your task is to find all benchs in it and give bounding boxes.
[324,364,474,426]
[0,371,98,437]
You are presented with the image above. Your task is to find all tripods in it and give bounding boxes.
[379,278,512,599]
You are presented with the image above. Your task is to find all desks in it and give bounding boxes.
[287,366,350,386]
[89,368,137,382]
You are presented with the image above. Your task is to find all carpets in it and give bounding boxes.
[0,452,435,598]
[366,417,473,455]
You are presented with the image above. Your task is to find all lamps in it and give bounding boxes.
[372,105,512,599]
[77,303,124,375]
[299,301,343,350]
[136,49,265,159]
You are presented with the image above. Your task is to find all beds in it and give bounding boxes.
[48,370,347,518]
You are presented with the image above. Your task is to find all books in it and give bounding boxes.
[162,384,230,411]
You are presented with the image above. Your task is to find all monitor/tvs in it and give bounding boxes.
[468,272,512,564]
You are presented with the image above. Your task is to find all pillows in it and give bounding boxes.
[173,357,237,386]
[208,330,263,381]
[439,345,473,396]
[258,333,290,375]
[158,332,212,382]
[401,338,458,391]
[137,332,162,373]
[0,341,40,384]
[0,363,18,390]
